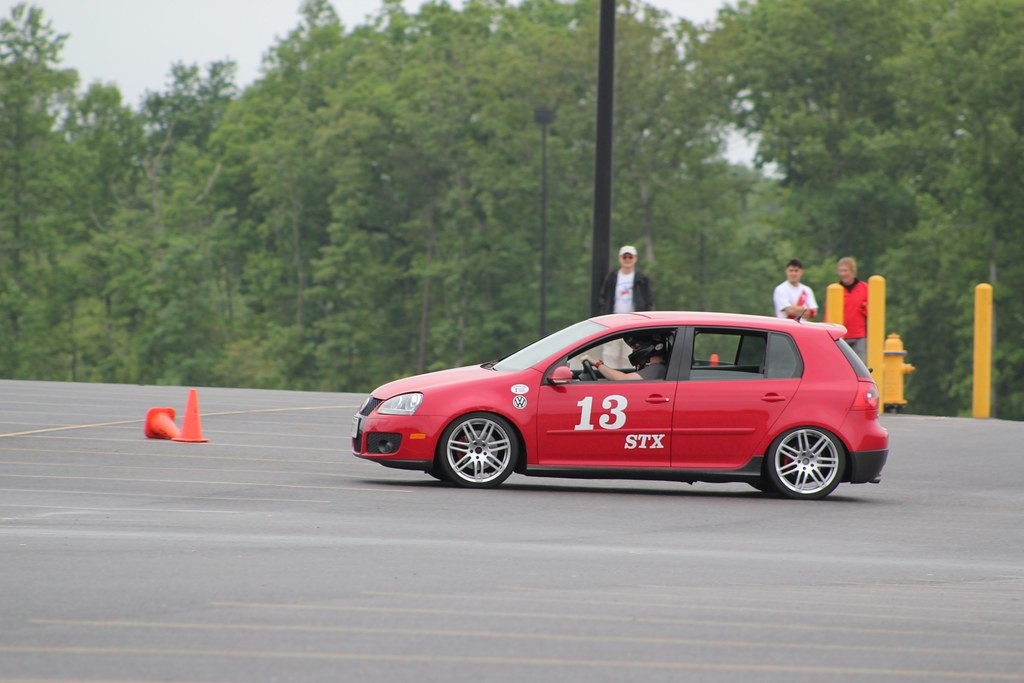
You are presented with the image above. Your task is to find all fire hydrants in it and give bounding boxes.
[881,332,915,414]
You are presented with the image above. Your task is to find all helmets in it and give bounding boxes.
[623,331,666,367]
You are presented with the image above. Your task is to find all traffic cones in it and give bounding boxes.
[145,407,180,441]
[170,386,212,444]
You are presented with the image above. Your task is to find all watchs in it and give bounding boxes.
[595,360,604,368]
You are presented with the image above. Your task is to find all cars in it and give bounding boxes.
[349,310,888,499]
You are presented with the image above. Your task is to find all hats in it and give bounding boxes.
[618,245,637,256]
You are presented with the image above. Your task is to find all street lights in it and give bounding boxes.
[533,102,553,339]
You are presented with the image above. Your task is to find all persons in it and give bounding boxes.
[773,259,818,321]
[825,257,867,362]
[581,328,672,380]
[600,245,652,369]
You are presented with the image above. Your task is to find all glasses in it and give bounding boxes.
[631,346,640,349]
[622,256,633,259]
